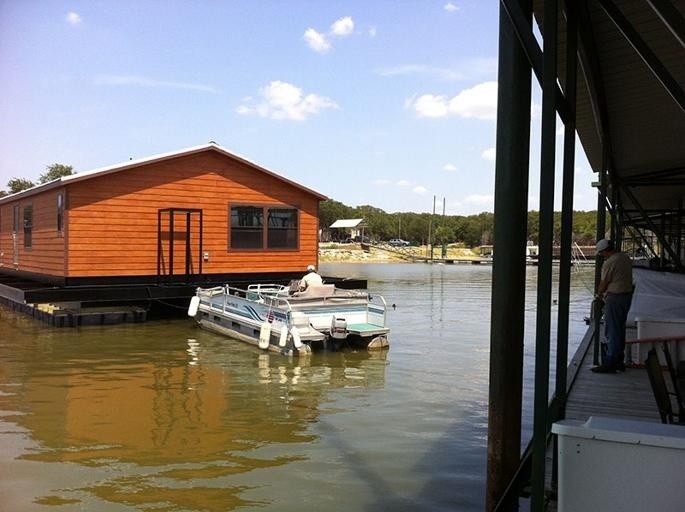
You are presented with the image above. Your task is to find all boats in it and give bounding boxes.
[186,280,391,358]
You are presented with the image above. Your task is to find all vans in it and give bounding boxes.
[388,238,411,247]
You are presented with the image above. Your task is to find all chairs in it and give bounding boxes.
[291,285,334,299]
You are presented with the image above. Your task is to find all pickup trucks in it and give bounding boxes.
[346,235,377,245]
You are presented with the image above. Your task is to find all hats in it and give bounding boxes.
[307,265,315,270]
[595,239,612,257]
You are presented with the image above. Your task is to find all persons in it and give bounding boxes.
[595,239,633,372]
[291,265,323,296]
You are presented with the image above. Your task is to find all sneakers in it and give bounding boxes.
[616,368,626,373]
[592,365,616,373]
